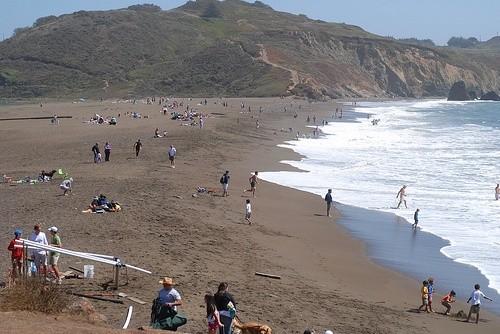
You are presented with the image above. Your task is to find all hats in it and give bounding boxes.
[158,277,175,285]
[47,227,58,232]
[15,230,23,235]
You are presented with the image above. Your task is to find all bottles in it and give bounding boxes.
[31,263,37,276]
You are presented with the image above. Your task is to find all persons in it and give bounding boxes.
[395,184,407,209]
[411,208,420,230]
[3,96,380,214]
[203,282,237,334]
[149,277,183,331]
[244,198,253,226]
[441,290,457,317]
[495,183,500,200]
[465,284,492,323]
[133,139,142,158]
[47,226,63,285]
[27,224,49,281]
[325,188,333,218]
[415,278,436,313]
[168,144,176,169]
[7,230,27,278]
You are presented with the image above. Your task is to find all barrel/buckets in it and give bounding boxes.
[83,264,94,278]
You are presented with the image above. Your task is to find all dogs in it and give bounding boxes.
[230,315,272,334]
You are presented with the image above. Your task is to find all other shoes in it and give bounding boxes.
[56,280,61,284]
[51,279,57,283]
